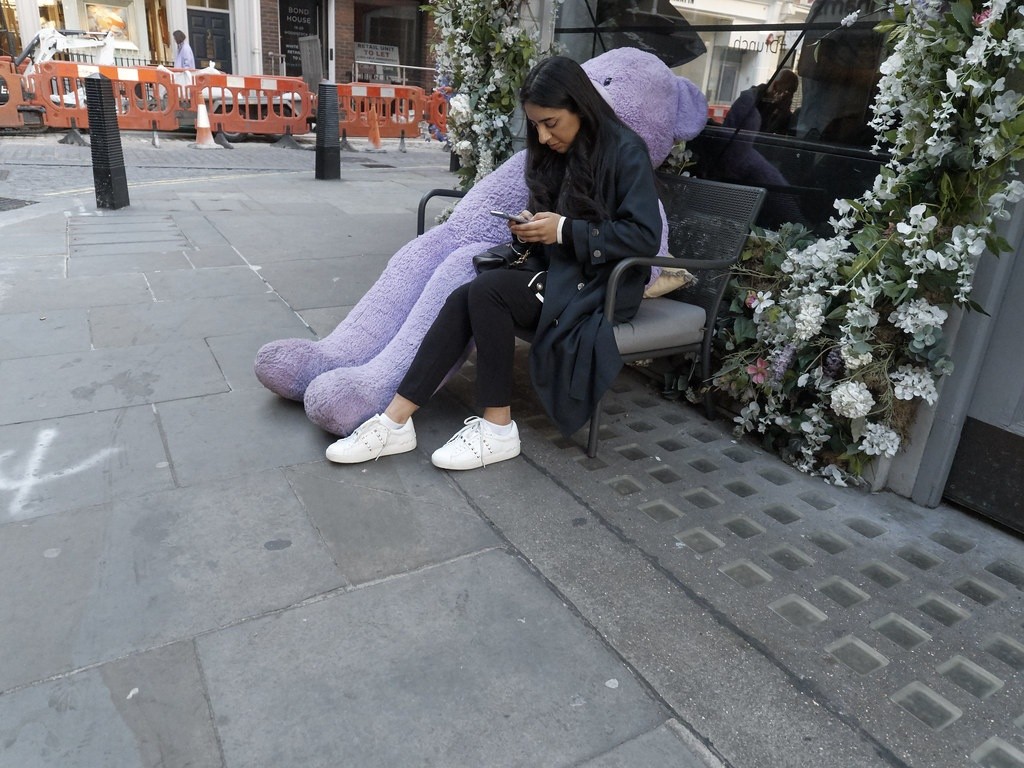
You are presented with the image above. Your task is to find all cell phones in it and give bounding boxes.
[489,210,529,223]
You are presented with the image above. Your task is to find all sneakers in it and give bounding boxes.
[325,413,417,463]
[431,415,521,470]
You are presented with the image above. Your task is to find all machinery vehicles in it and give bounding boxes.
[0,30,117,137]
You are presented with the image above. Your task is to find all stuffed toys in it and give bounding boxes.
[253,40,708,436]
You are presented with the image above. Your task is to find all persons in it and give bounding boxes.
[168,30,196,73]
[326,55,663,470]
[720,70,800,132]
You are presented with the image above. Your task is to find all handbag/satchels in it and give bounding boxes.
[473,241,543,276]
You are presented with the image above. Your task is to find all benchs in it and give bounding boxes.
[417,169,768,459]
